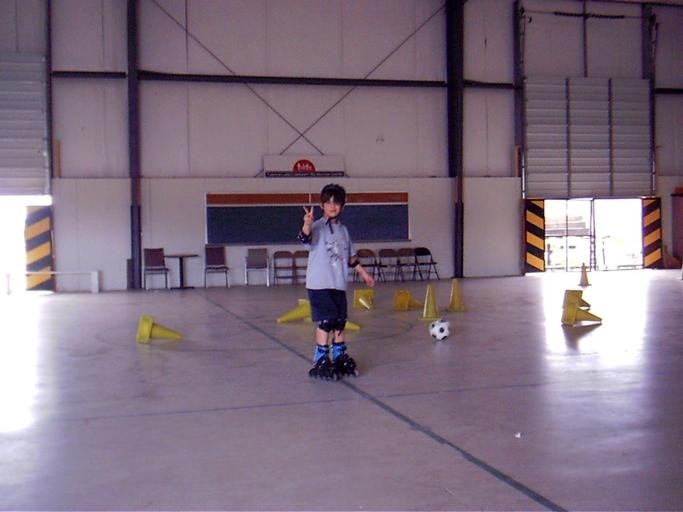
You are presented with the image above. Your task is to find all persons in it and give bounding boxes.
[298,184,375,375]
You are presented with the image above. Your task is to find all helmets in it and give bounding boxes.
[321,184,346,204]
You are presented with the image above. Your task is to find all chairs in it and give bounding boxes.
[140,248,170,290]
[203,247,229,288]
[377,250,403,283]
[245,248,271,287]
[414,248,440,282]
[356,249,384,283]
[398,249,424,283]
[295,251,309,286]
[273,251,295,286]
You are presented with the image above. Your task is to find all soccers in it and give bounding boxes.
[429,319,451,341]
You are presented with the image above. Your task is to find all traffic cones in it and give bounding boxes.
[559,289,591,310]
[444,277,466,313]
[351,287,377,312]
[270,297,310,326]
[345,320,361,333]
[558,300,602,325]
[416,282,442,324]
[389,288,423,312]
[132,313,182,346]
[575,260,591,286]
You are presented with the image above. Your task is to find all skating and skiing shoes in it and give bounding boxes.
[333,345,359,379]
[308,344,338,381]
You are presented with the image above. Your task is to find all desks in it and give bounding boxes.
[164,255,199,289]
